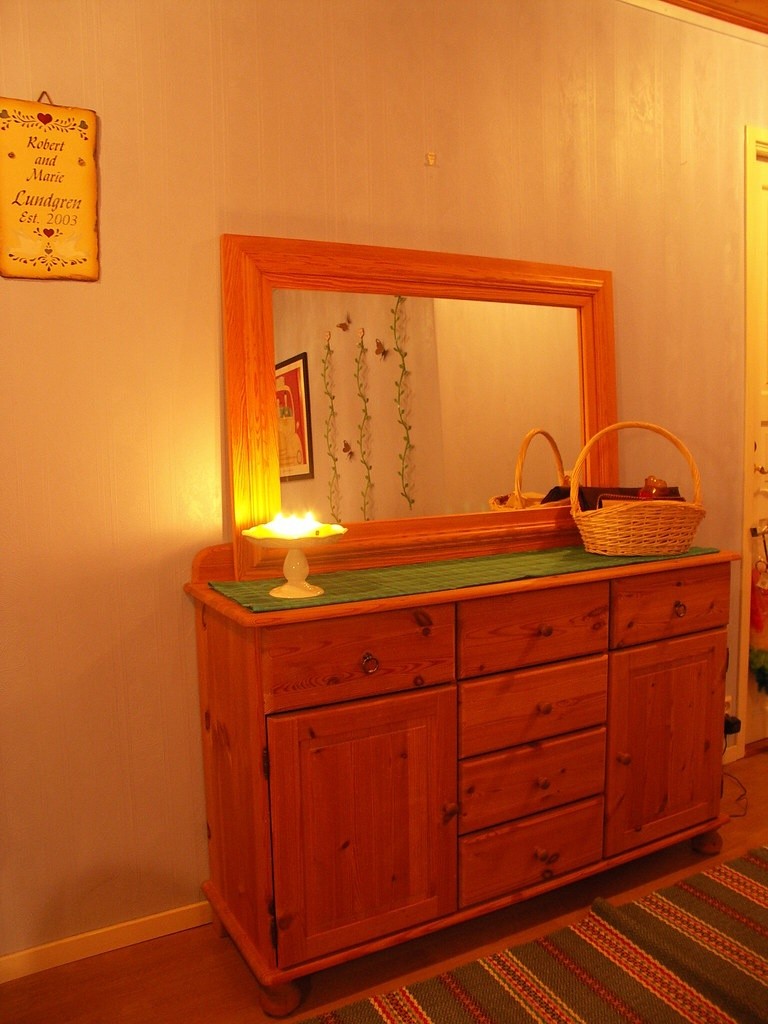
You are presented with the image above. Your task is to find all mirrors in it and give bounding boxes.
[219,231,620,582]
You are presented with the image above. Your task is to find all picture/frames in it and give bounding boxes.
[274,352,316,482]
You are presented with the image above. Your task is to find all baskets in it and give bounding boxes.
[569,421,706,556]
[488,430,569,509]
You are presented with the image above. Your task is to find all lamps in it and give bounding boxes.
[240,509,348,599]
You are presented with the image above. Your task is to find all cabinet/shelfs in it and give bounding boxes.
[187,541,737,1021]
